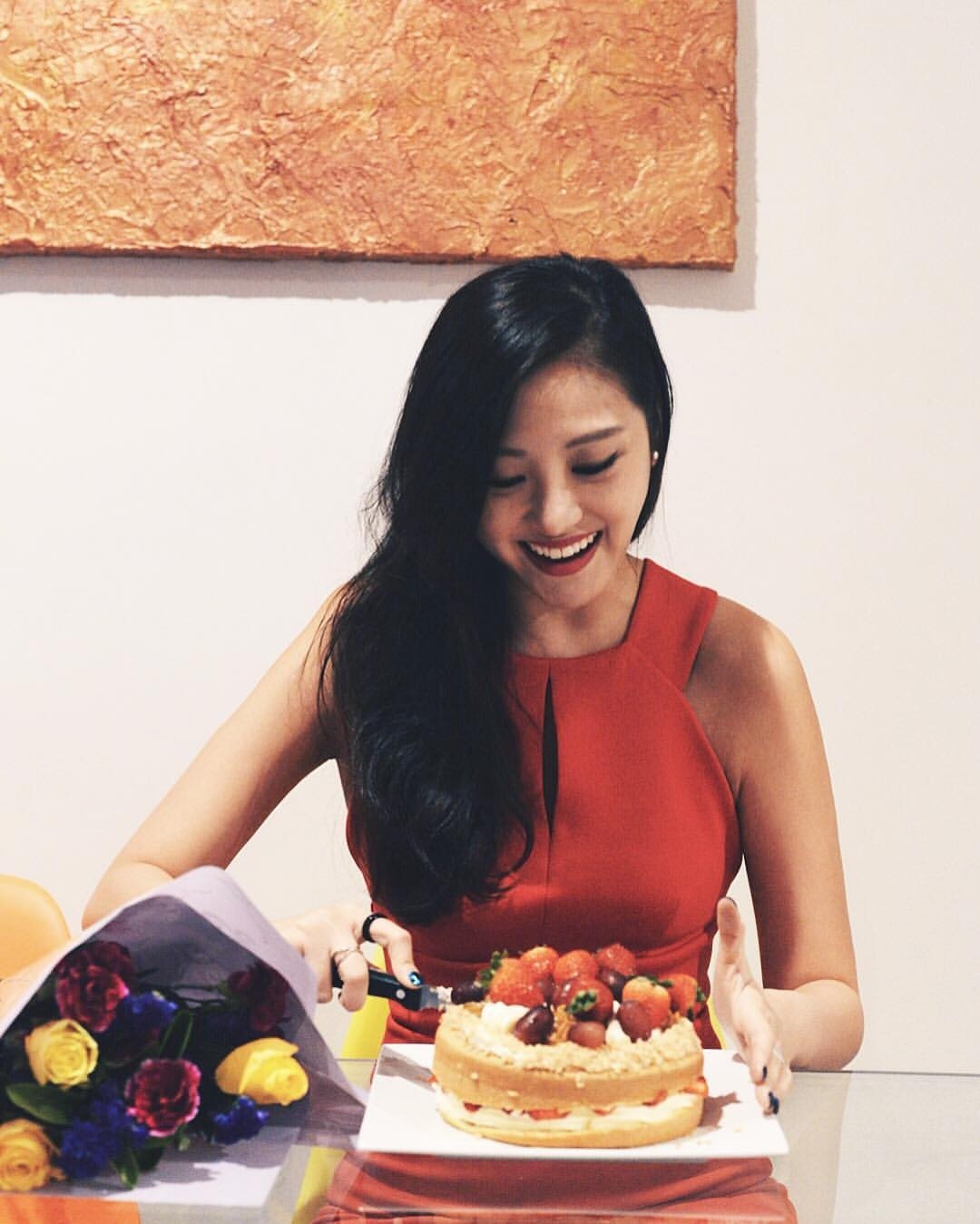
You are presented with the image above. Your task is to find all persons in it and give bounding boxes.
[81,253,866,1223]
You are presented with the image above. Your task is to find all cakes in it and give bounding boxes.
[432,945,707,1149]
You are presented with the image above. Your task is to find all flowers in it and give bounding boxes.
[0,865,367,1195]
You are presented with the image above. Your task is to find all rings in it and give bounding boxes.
[362,913,387,943]
[328,938,364,966]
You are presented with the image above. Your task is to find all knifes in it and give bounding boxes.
[329,966,453,1012]
